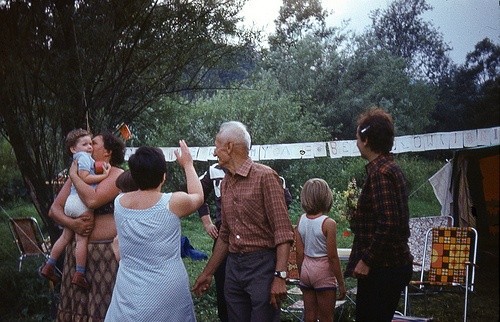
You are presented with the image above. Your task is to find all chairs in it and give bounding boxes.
[404,214,478,322]
[8,217,50,272]
[280,278,358,322]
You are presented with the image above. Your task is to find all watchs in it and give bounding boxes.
[273,270,287,280]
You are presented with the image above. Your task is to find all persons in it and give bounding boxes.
[197,123,293,322]
[47,130,126,322]
[342,110,414,322]
[190,120,294,322]
[112,168,140,264]
[296,178,346,322]
[102,140,204,322]
[41,128,112,290]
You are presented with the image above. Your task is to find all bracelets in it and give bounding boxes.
[337,279,343,283]
